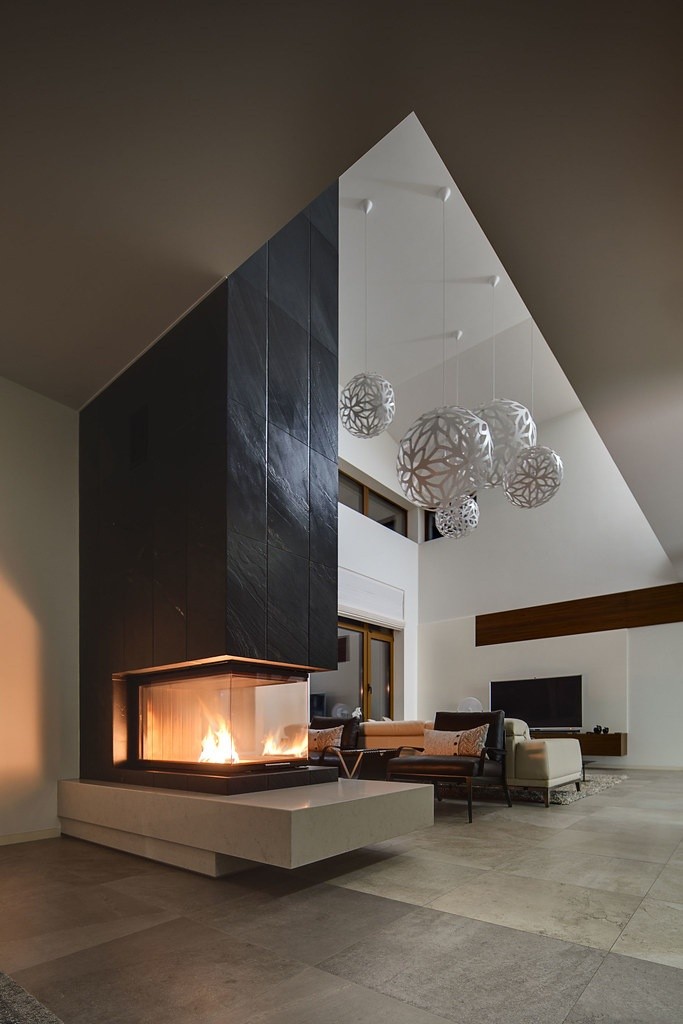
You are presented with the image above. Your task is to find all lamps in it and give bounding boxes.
[396,185,565,539]
[340,198,396,438]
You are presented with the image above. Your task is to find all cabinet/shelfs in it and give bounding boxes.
[530,731,627,757]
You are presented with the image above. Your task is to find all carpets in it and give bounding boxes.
[432,774,628,807]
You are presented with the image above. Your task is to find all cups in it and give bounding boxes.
[603,728,607,733]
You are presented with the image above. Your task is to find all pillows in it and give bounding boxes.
[308,725,344,754]
[421,723,490,759]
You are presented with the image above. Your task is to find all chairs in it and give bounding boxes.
[385,709,514,822]
[308,716,358,779]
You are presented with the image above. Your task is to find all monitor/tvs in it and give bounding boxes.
[490,675,583,731]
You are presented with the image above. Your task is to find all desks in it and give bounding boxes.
[327,747,401,779]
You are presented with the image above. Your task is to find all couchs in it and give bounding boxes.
[358,717,584,809]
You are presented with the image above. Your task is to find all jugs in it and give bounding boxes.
[594,725,601,733]
